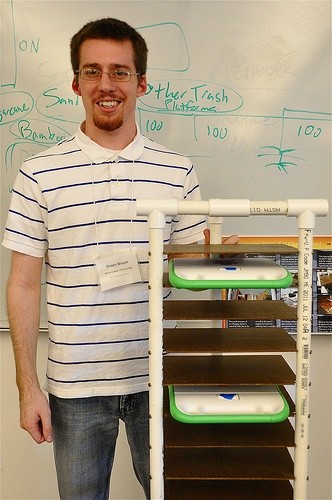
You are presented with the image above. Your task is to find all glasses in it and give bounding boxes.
[75,66,141,82]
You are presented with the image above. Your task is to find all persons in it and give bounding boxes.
[2,18,240,500]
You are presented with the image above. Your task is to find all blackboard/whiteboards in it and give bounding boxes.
[0,1,332,335]
